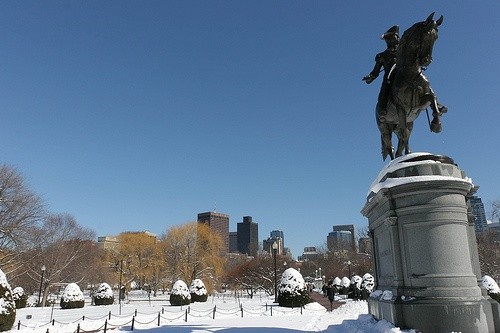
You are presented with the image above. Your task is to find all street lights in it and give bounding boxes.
[272,241,279,303]
[348,260,351,278]
[37,265,46,307]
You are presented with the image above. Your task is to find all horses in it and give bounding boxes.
[375,11,447,159]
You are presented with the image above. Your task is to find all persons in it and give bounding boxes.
[322,284,326,296]
[362,24,448,114]
